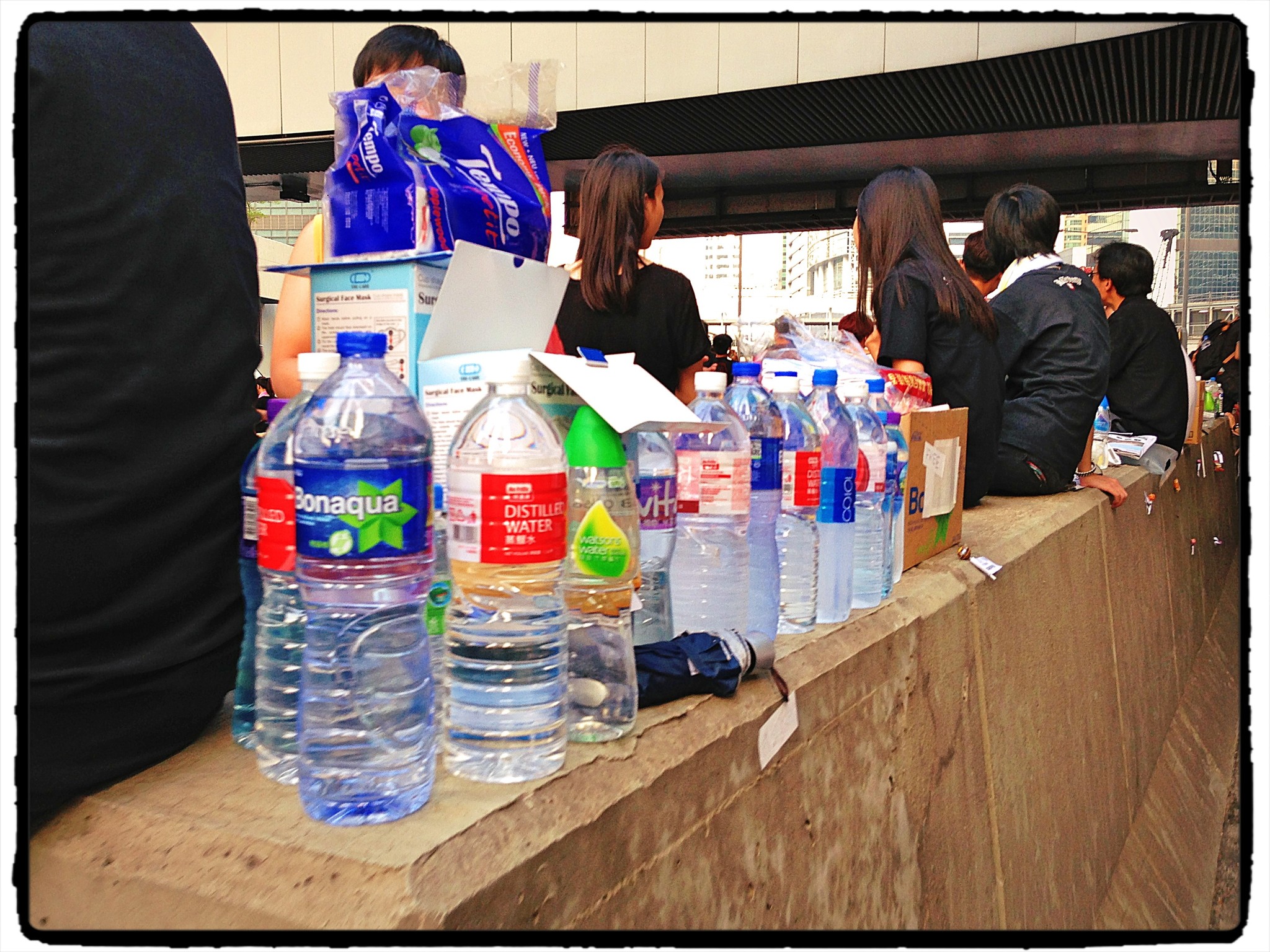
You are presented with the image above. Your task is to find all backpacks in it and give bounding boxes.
[1192,316,1241,380]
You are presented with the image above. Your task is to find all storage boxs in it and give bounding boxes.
[1185,379,1206,444]
[903,406,967,572]
[309,239,728,493]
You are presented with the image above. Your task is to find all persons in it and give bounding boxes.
[269,27,472,398]
[834,311,874,355]
[954,230,1004,299]
[543,148,717,402]
[1088,241,1196,470]
[849,165,1000,512]
[982,184,1129,510]
[760,316,802,363]
[1188,302,1240,436]
[711,334,740,387]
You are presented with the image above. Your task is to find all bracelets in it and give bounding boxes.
[1075,462,1097,476]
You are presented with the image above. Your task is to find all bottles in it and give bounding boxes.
[1203,377,1223,413]
[231,332,910,827]
[1091,395,1111,469]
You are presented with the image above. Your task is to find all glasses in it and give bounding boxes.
[1087,272,1099,279]
